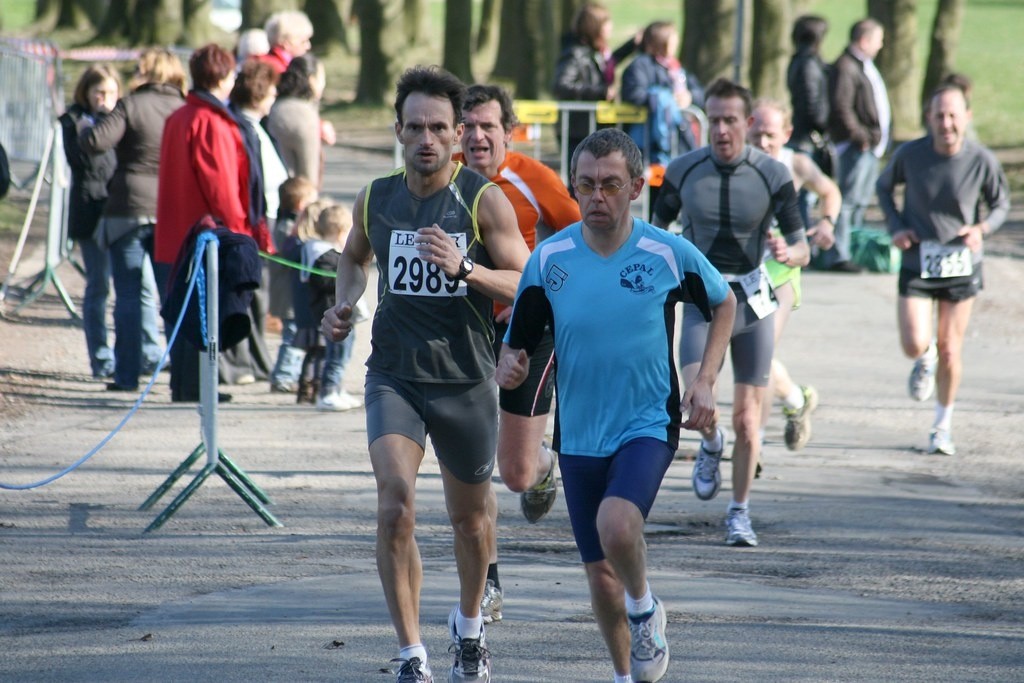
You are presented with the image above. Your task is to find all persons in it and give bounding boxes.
[58,63,170,378]
[829,20,891,272]
[651,77,810,545]
[948,75,970,91]
[495,128,736,683]
[876,85,1010,456]
[321,65,532,683]
[236,9,336,187]
[154,44,277,404]
[218,60,291,386]
[269,176,370,409]
[552,4,704,226]
[77,49,188,391]
[748,98,842,479]
[451,85,583,623]
[787,16,834,225]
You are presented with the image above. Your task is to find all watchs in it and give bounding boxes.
[454,256,474,280]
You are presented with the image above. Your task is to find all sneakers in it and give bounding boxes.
[521,433,556,523]
[692,429,724,501]
[783,384,819,452]
[626,593,670,682]
[926,423,955,456]
[318,387,362,411]
[483,578,504,625]
[447,604,492,682]
[907,347,940,401]
[724,501,760,546]
[390,656,434,683]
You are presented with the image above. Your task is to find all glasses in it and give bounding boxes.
[574,182,633,197]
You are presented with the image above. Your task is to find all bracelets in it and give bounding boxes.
[825,216,837,224]
[784,257,789,263]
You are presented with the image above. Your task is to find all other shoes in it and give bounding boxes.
[174,386,233,404]
[296,378,316,405]
[271,372,296,395]
[102,376,137,390]
